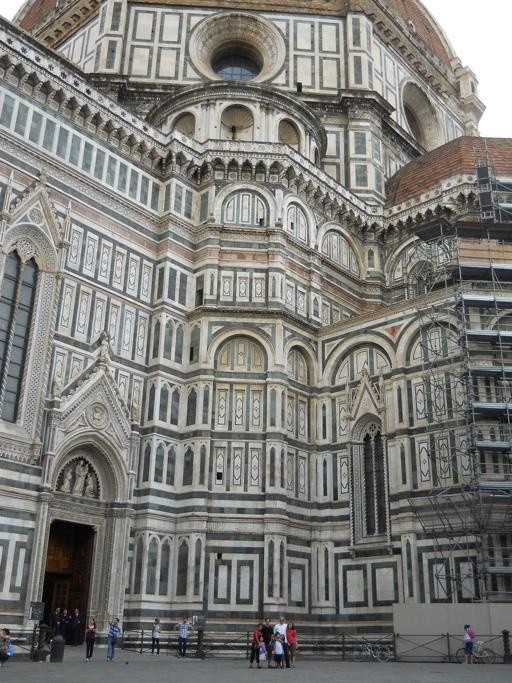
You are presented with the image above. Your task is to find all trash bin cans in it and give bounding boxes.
[50,635,64,663]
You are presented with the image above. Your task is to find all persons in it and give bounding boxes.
[176,616,194,658]
[60,460,95,496]
[151,618,160,656]
[250,617,296,669]
[54,607,81,646]
[0,628,10,663]
[464,624,474,664]
[106,617,121,662]
[85,618,96,661]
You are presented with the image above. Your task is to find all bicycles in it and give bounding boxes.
[350,635,395,663]
[454,636,495,664]
